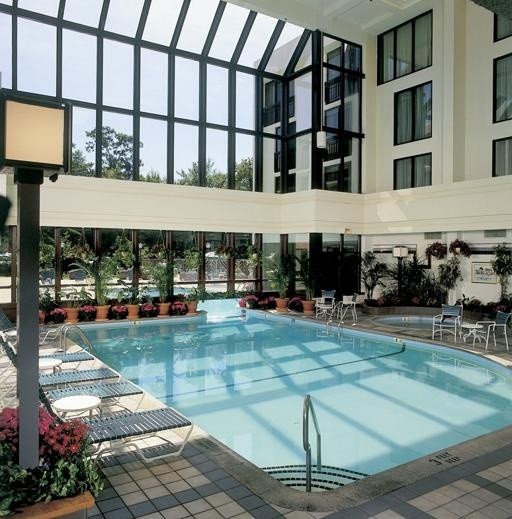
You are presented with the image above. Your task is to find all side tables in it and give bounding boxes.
[462,323,483,344]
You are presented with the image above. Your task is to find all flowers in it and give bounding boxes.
[0,406,104,519]
[239,295,304,312]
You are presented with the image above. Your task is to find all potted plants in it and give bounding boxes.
[38,232,215,323]
[294,252,316,315]
[267,254,296,313]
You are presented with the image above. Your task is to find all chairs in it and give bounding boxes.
[339,291,360,323]
[315,288,336,318]
[473,310,512,351]
[0,309,194,468]
[432,303,463,344]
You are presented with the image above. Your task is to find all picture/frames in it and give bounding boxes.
[471,261,498,284]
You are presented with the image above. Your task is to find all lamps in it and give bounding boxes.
[316,28,326,148]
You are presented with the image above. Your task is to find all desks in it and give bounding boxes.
[10,490,95,519]
